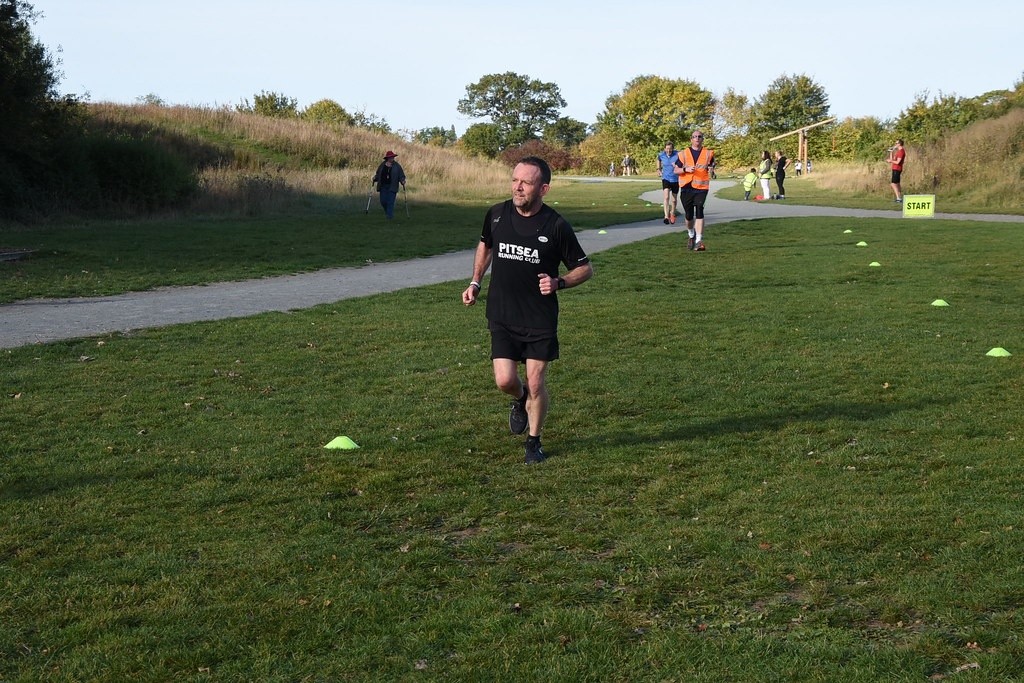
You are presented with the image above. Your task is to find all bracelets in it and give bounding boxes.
[470,281,482,291]
[657,168,661,171]
[683,168,686,173]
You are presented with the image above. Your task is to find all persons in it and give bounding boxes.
[711,162,716,179]
[656,142,681,225]
[373,151,406,219]
[886,139,906,203]
[743,168,757,201]
[758,151,772,201]
[774,151,791,199]
[674,131,716,251]
[609,155,637,177]
[462,157,594,465]
[795,158,811,176]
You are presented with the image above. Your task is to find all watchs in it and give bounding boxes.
[557,277,566,291]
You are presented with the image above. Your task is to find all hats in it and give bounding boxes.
[383,151,398,159]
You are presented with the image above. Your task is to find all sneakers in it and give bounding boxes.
[694,241,705,251]
[509,385,528,435]
[688,228,696,249]
[525,435,545,466]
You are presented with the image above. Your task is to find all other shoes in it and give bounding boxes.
[670,210,675,224]
[664,218,669,224]
[895,199,902,203]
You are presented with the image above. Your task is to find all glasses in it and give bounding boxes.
[691,135,702,138]
